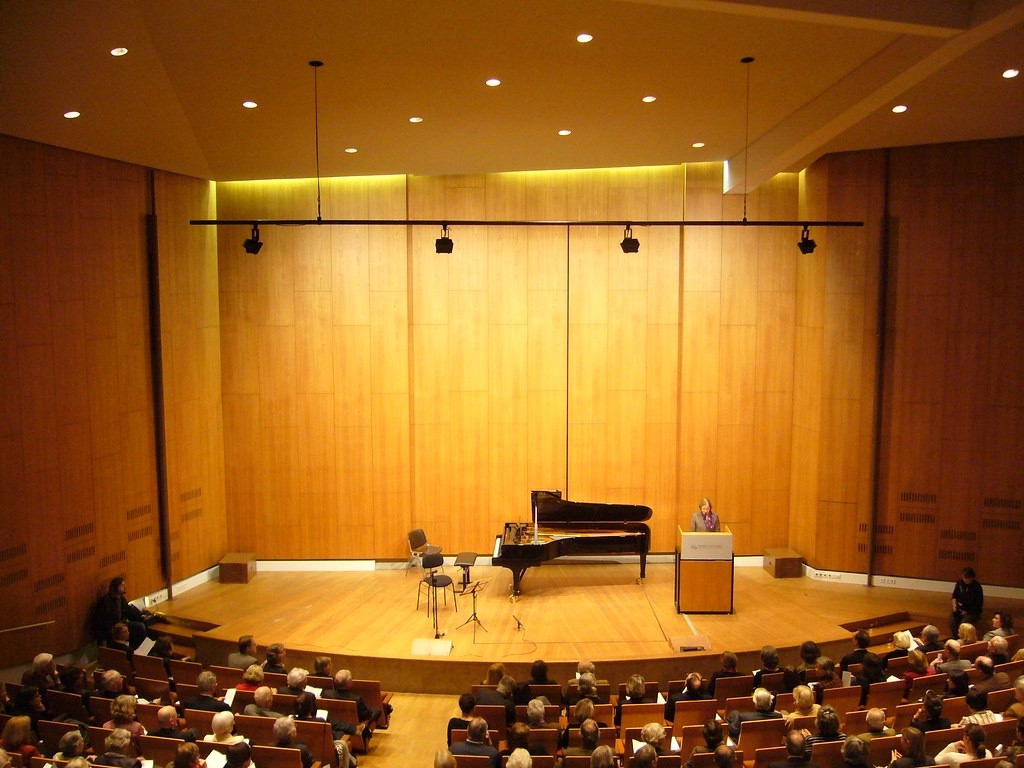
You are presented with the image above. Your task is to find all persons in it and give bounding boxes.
[949,567,984,639]
[0,622,392,768]
[94,578,145,643]
[690,498,721,533]
[434,609,1024,768]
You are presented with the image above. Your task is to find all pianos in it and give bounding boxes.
[492,489,653,603]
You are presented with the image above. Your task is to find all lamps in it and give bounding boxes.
[621,228,640,253]
[436,230,454,254]
[799,227,817,255]
[244,228,263,253]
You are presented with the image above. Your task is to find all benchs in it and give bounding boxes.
[454,551,477,593]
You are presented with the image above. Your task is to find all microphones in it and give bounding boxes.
[513,615,523,627]
[427,570,438,575]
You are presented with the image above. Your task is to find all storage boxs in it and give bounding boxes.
[763,548,802,577]
[218,552,256,583]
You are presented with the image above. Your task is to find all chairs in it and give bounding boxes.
[452,634,1024,768]
[405,529,444,578]
[416,553,457,617]
[1,647,389,768]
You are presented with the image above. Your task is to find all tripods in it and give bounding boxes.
[455,582,488,644]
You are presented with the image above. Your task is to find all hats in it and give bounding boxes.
[287,667,310,685]
